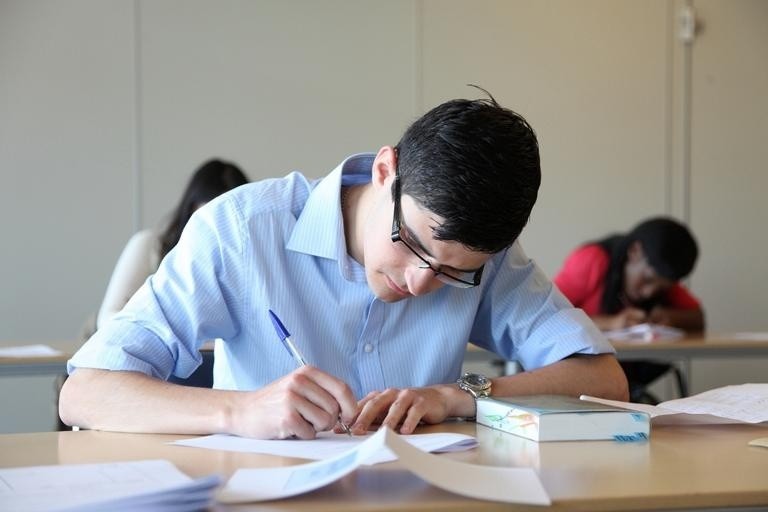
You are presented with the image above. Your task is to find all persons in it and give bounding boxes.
[94,158,251,334]
[56,83,631,440]
[552,215,708,403]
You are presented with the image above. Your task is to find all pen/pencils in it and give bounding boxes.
[268,308,352,438]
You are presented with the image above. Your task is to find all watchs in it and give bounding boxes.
[452,370,500,421]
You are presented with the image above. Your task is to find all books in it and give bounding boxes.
[1,458,221,511]
[476,395,652,442]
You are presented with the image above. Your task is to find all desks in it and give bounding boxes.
[0,340,98,377]
[0,412,767,512]
[604,331,767,357]
[460,342,519,376]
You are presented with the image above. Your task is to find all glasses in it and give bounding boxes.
[392,147,484,289]
